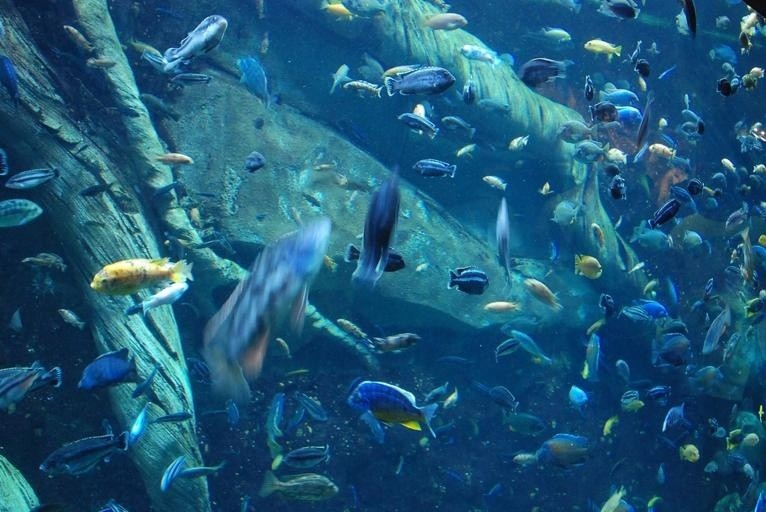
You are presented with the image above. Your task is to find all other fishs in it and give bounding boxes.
[0,0,766,512]
